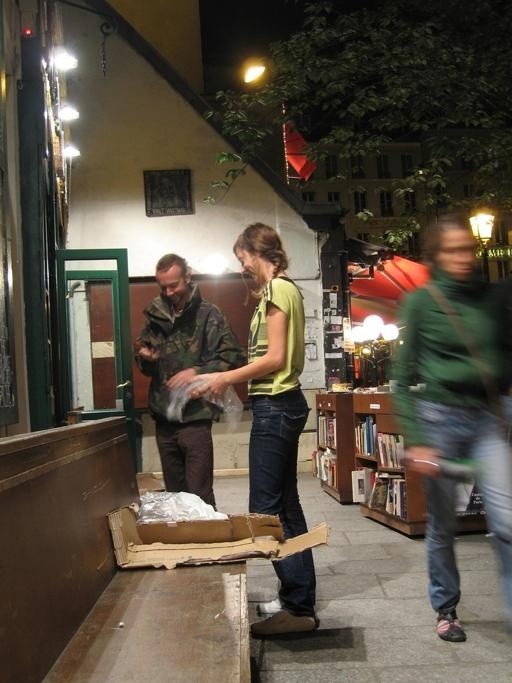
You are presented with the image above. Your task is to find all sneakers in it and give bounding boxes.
[252,610,316,635]
[256,599,284,613]
[437,611,466,641]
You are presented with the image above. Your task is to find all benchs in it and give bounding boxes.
[0,416,252,683]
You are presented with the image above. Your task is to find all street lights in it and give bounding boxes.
[467,204,502,283]
[351,314,400,387]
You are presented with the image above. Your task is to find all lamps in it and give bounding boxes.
[349,314,406,387]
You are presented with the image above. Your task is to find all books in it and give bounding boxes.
[313,416,407,520]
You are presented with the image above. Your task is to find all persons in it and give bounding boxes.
[389,213,512,643]
[188,223,320,638]
[134,253,239,512]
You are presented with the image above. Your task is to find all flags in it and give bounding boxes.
[285,111,317,182]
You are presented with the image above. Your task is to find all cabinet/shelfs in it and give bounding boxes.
[353,394,488,536]
[316,392,355,503]
